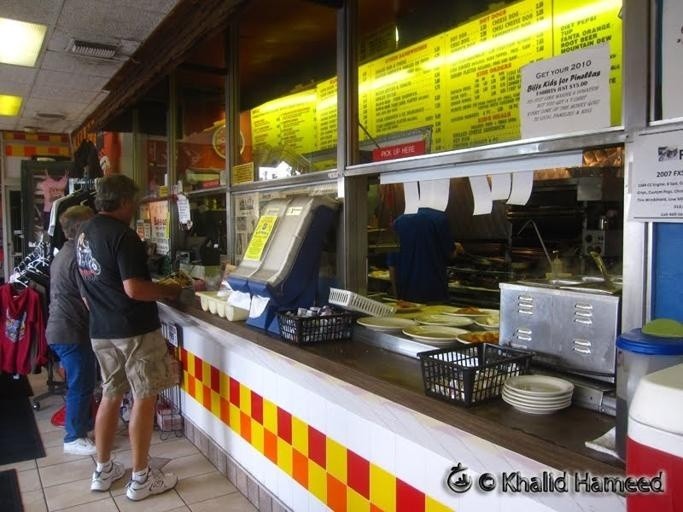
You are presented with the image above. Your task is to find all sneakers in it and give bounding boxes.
[63,436,97,455]
[90,460,125,492]
[87,430,95,441]
[125,469,178,501]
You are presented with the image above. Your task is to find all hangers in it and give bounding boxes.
[8,240,50,288]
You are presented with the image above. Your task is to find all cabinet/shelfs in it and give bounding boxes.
[155,289,629,512]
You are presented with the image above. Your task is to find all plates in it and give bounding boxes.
[402,326,468,347]
[502,373,575,416]
[383,302,422,313]
[474,317,500,331]
[356,315,415,331]
[457,331,476,344]
[417,315,472,326]
[449,308,499,317]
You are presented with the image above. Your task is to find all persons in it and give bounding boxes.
[75,172,184,502]
[386,208,464,305]
[43,205,98,458]
